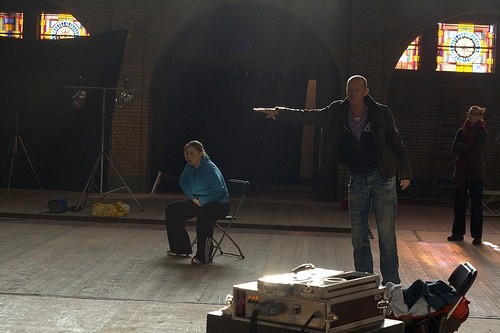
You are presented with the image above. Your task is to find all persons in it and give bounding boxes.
[252,75,412,288]
[165,140,230,264]
[447,105,489,245]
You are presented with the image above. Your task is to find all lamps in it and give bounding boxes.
[115,90,135,108]
[70,88,88,110]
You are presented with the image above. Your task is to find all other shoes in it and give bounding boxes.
[167,250,178,256]
[191,256,203,265]
[472,238,481,245]
[448,234,464,241]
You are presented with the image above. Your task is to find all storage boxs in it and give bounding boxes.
[206,306,405,333]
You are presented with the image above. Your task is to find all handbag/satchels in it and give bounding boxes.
[92,201,130,218]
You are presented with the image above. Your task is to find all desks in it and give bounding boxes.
[481,190,500,216]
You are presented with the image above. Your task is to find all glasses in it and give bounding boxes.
[467,112,481,116]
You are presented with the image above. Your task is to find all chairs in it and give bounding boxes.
[184,176,252,263]
[406,262,478,333]
